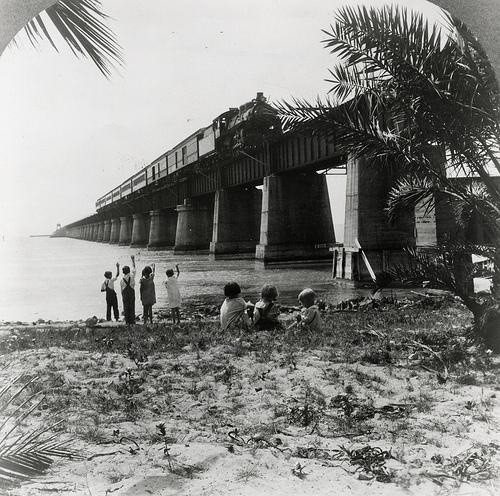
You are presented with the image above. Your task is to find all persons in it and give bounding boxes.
[286,288,322,330]
[139,262,156,323]
[100,262,119,321]
[220,280,254,329]
[253,284,282,331]
[120,255,137,323]
[163,262,183,323]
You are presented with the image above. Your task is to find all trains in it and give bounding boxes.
[96,92,283,212]
